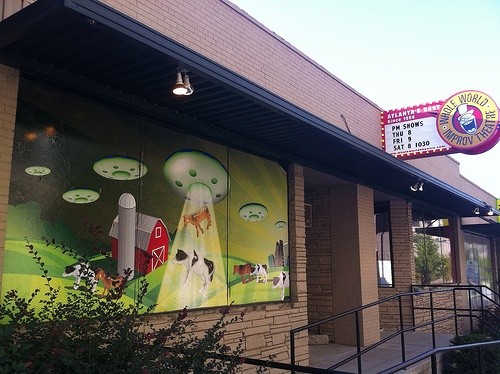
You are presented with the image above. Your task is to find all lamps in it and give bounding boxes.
[472,206,480,215]
[410,179,424,192]
[173,67,195,96]
[487,206,494,215]
